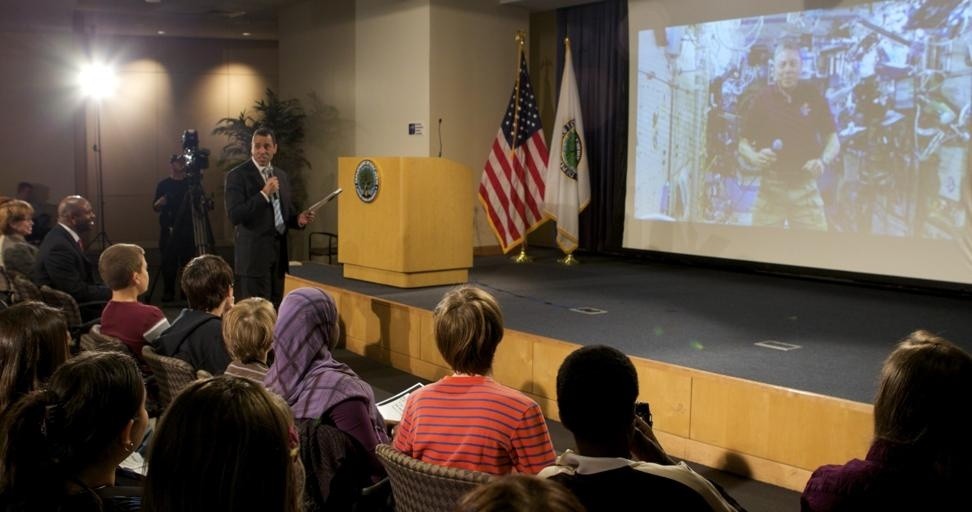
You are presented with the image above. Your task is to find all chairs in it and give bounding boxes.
[142,344,195,411]
[13,275,41,301]
[374,443,501,512]
[41,286,107,353]
[89,324,160,416]
[0,266,14,308]
[309,230,338,263]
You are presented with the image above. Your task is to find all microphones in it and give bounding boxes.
[266,167,278,200]
[771,139,783,156]
[438,118,442,156]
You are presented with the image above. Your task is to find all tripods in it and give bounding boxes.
[145,175,217,306]
[83,112,113,251]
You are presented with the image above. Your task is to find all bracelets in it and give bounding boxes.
[818,157,829,170]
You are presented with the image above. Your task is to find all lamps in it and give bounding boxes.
[78,62,119,236]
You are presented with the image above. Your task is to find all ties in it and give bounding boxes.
[263,170,285,235]
[78,239,85,254]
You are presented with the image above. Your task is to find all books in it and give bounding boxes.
[306,188,342,216]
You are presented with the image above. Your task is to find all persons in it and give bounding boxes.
[390,286,556,477]
[0,200,43,283]
[29,195,112,302]
[0,301,72,409]
[799,328,970,511]
[533,344,746,512]
[221,296,278,387]
[225,127,317,310]
[97,243,172,375]
[267,286,388,481]
[442,473,585,512]
[156,253,234,375]
[0,350,150,511]
[738,40,841,232]
[142,374,307,511]
[153,153,206,304]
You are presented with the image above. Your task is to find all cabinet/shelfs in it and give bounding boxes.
[635,30,714,219]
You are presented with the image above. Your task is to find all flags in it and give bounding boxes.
[542,40,592,256]
[477,44,553,255]
